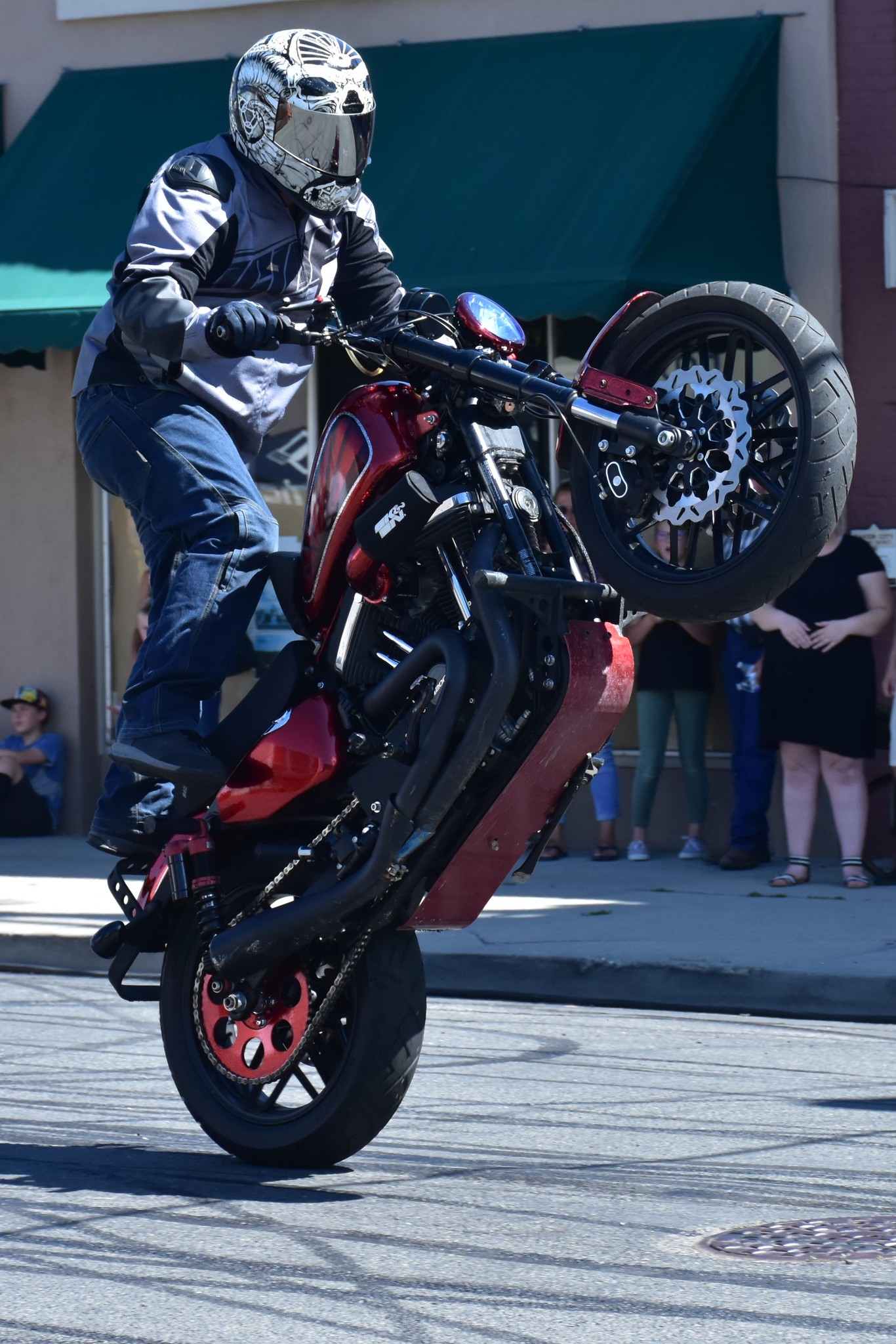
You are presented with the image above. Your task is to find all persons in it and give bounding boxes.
[70,23,463,861]
[0,684,64,836]
[531,480,623,862]
[715,452,896,886]
[625,513,711,859]
[747,509,893,889]
[881,634,896,788]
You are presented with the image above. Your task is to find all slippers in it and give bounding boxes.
[593,844,618,861]
[539,845,568,861]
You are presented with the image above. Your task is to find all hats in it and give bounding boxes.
[0,686,48,711]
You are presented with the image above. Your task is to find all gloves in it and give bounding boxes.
[205,298,293,358]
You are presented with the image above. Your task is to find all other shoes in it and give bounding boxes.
[707,846,773,870]
[627,840,650,860]
[110,729,254,784]
[86,828,163,859]
[678,836,707,858]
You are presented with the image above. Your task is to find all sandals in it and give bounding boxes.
[770,854,810,885]
[840,857,870,889]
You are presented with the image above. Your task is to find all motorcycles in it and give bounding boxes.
[86,279,857,1170]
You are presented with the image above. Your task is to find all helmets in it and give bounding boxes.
[229,29,377,212]
